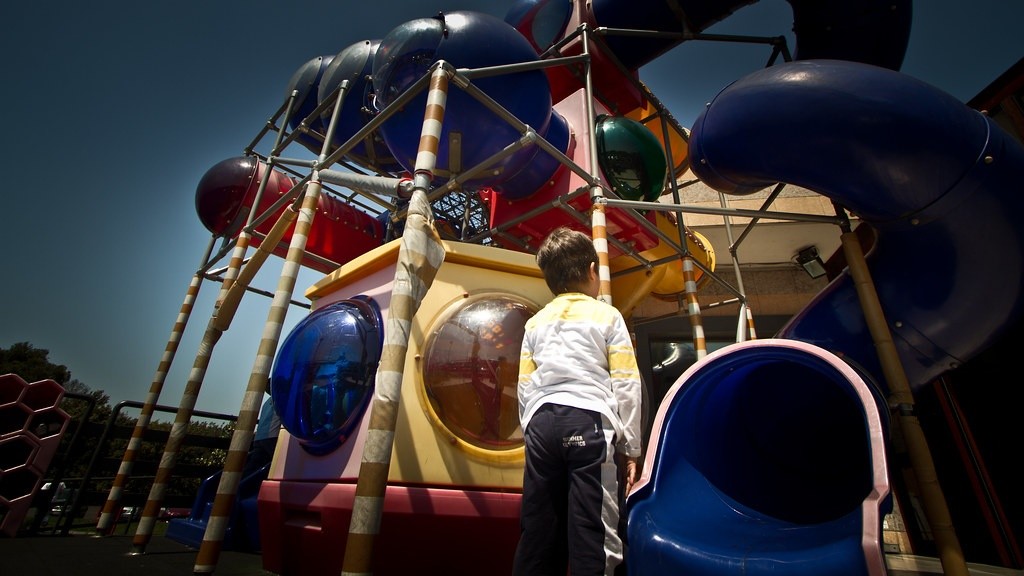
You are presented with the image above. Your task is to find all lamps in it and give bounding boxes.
[792,245,830,280]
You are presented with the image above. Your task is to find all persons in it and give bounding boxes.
[511,226,642,576]
[494,356,507,419]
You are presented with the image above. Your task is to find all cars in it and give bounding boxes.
[118,505,144,523]
[53,491,90,519]
[159,507,192,522]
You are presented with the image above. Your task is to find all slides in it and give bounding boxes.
[519,1,1022,576]
